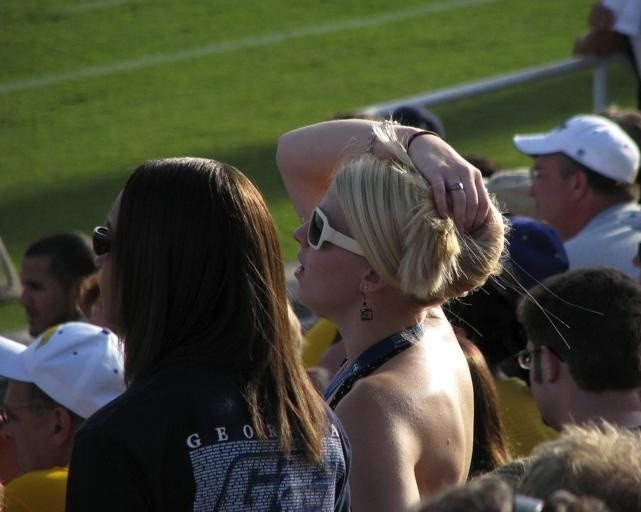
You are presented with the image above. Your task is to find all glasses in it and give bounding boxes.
[1,405,62,424]
[92,226,119,254]
[516,346,565,369]
[307,204,367,256]
[527,163,567,182]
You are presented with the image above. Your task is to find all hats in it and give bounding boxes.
[513,113,640,184]
[0,320,128,420]
[482,167,574,241]
[500,217,569,289]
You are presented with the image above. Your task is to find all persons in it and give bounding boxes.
[0,157,351,512]
[274,105,641,512]
[572,0,640,112]
